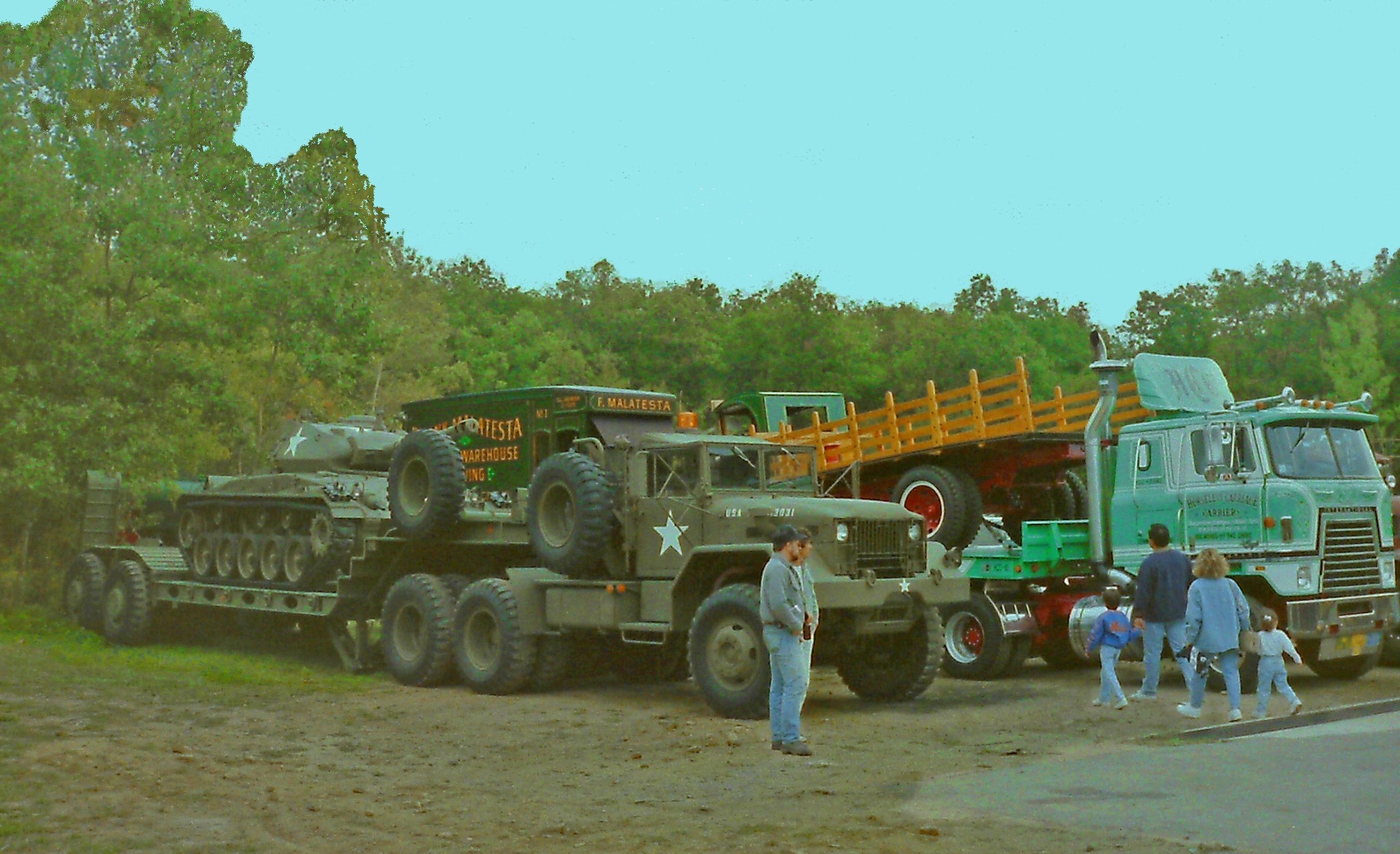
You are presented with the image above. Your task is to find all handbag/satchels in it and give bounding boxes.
[1237,627,1261,654]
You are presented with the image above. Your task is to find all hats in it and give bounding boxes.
[772,524,808,543]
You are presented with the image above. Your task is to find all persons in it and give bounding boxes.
[760,524,820,756]
[1084,588,1143,710]
[1129,523,1196,702]
[1176,547,1255,722]
[1251,608,1303,719]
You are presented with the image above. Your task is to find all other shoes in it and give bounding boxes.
[1094,699,1109,707]
[1229,709,1242,722]
[771,739,782,750]
[1114,698,1128,709]
[1251,711,1266,719]
[781,740,812,755]
[799,735,806,742]
[1131,691,1157,702]
[1291,700,1302,714]
[1177,704,1200,718]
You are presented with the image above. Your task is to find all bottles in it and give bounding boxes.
[804,613,811,640]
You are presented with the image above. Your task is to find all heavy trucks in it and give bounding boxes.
[709,331,1399,682]
[63,386,970,719]
